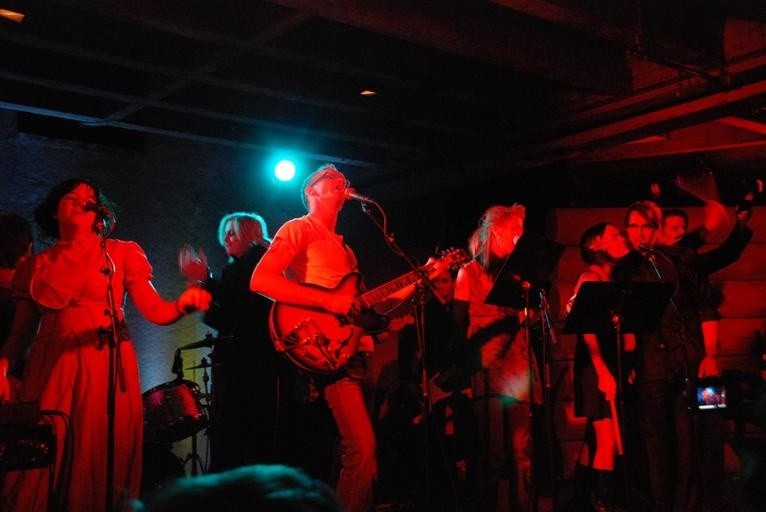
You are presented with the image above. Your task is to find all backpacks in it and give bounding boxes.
[141,379,208,443]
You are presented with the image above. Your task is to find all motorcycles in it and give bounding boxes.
[180,432,206,476]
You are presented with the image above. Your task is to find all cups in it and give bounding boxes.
[311,171,351,189]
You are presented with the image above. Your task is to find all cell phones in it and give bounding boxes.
[689,382,744,414]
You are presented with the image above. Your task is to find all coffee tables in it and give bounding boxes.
[187,361,221,370]
[179,333,234,350]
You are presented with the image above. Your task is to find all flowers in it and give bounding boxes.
[344,187,376,205]
[638,245,656,262]
[82,199,111,216]
[508,271,532,290]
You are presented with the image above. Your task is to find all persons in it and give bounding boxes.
[179,213,271,473]
[2,178,213,510]
[4,215,33,499]
[367,164,752,511]
[251,164,452,511]
